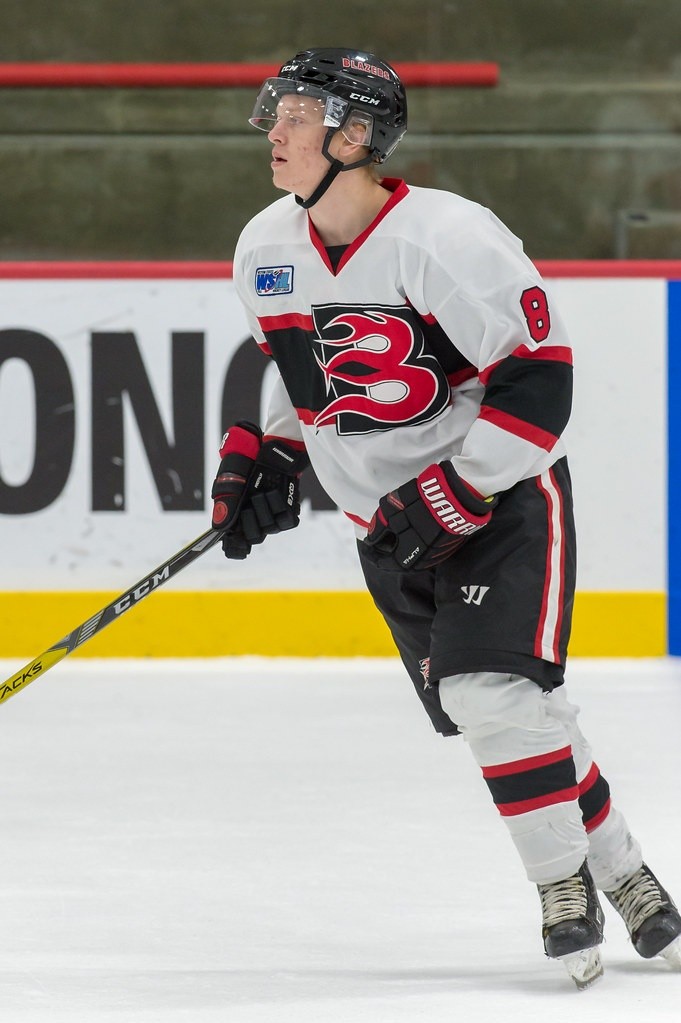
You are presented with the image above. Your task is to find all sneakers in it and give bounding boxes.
[537,858,605,991]
[603,860,681,972]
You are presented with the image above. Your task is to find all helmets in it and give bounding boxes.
[277,47,408,164]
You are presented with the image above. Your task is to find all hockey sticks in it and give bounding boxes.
[0,436,312,707]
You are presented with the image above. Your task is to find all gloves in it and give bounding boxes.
[213,420,309,558]
[365,460,498,574]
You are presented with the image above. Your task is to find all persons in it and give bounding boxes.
[209,43,681,992]
